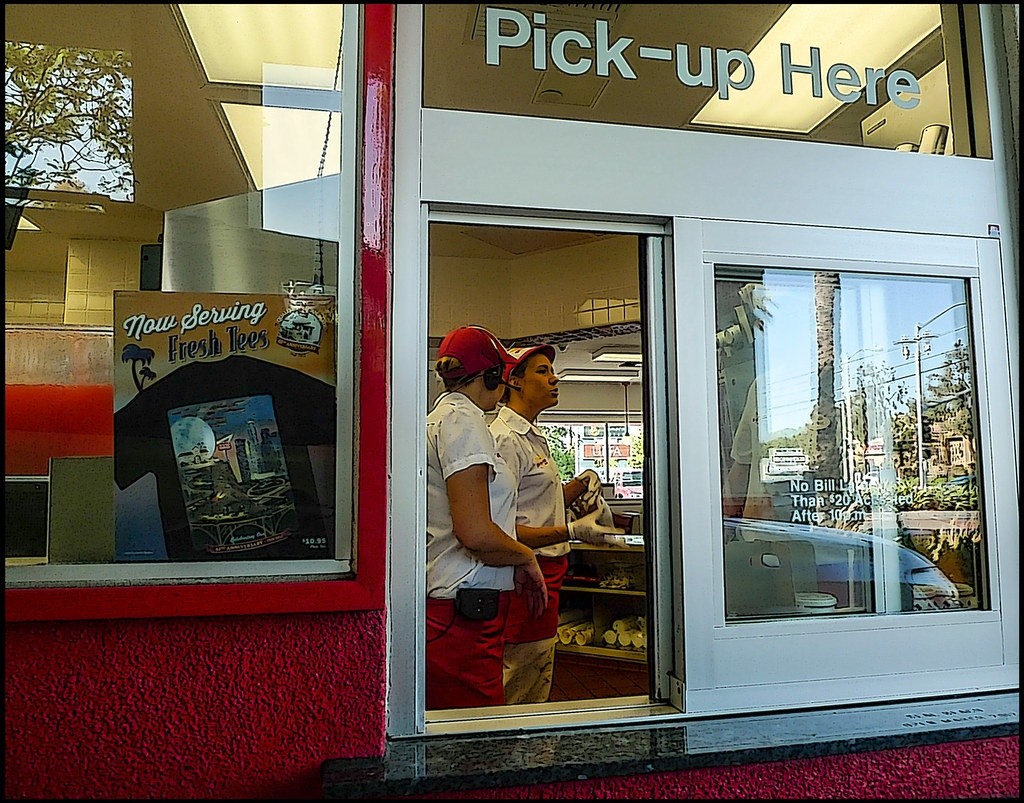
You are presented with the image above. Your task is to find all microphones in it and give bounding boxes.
[498,379,521,391]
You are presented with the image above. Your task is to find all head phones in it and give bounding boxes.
[483,331,504,391]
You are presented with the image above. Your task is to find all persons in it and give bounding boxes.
[488,340,632,706]
[425,324,547,711]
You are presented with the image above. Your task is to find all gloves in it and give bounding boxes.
[568,506,627,548]
[571,467,611,509]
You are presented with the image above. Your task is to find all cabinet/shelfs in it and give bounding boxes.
[555,543,647,666]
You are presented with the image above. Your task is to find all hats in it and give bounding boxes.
[505,345,557,380]
[438,324,521,372]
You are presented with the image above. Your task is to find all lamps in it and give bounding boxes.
[591,345,643,364]
[557,369,639,383]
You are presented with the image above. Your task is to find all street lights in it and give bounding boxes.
[892,325,940,490]
[843,345,883,488]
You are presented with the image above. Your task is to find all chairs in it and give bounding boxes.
[752,538,818,594]
[724,540,799,617]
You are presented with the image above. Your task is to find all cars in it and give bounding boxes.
[610,468,644,498]
[723,516,960,621]
